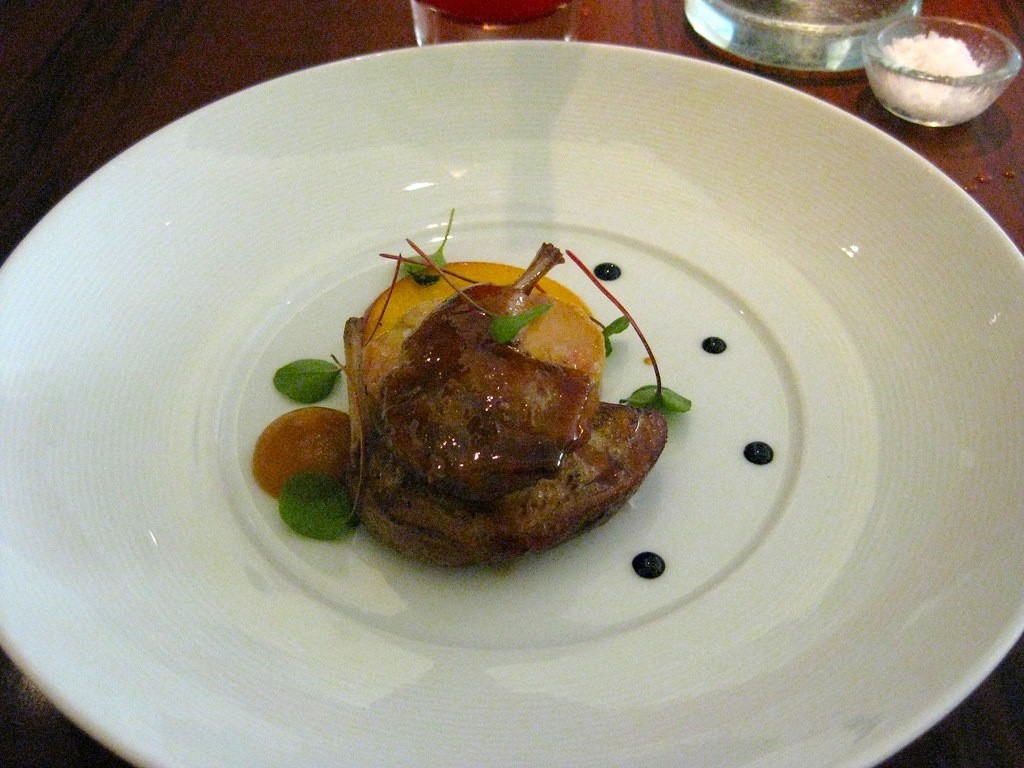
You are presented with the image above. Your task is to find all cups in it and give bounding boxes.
[407,0,584,47]
[682,0,924,75]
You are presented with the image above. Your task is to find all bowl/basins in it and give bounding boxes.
[861,17,1023,129]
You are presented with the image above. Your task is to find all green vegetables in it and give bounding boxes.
[269,210,693,541]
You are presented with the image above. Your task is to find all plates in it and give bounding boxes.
[2,39,1023,768]
